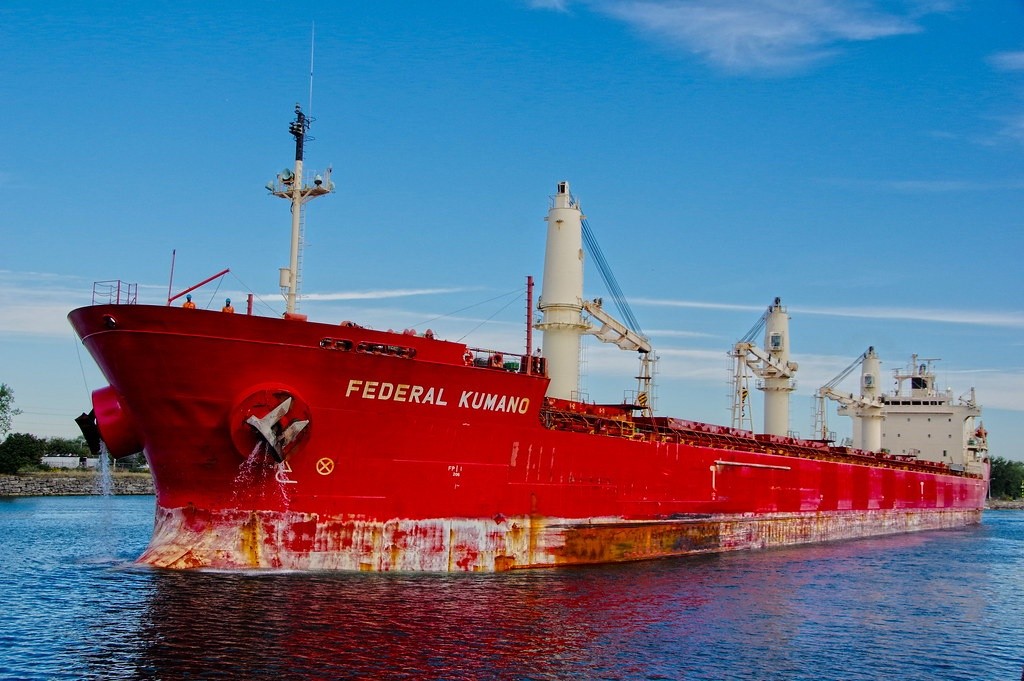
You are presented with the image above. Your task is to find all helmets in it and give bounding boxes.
[225,298,231,302]
[186,294,192,298]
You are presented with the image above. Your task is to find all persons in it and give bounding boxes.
[183,294,195,310]
[532,348,541,374]
[221,298,234,313]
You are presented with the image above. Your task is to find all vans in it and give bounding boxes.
[119,461,141,467]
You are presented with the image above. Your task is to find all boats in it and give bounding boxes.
[67,19,990,576]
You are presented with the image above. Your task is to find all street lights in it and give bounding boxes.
[989,477,995,499]
